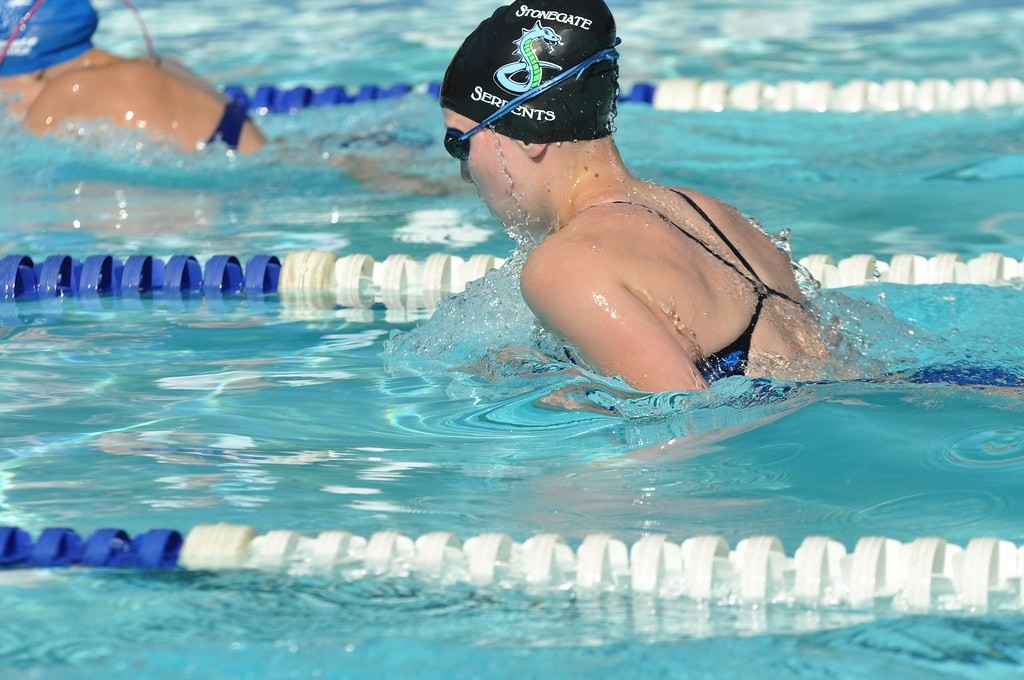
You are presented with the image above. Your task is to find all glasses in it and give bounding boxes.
[444,37,622,161]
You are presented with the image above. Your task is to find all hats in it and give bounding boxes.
[0,0,98,76]
[440,0,621,144]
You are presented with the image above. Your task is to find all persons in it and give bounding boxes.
[1,1,264,155]
[441,0,820,393]
